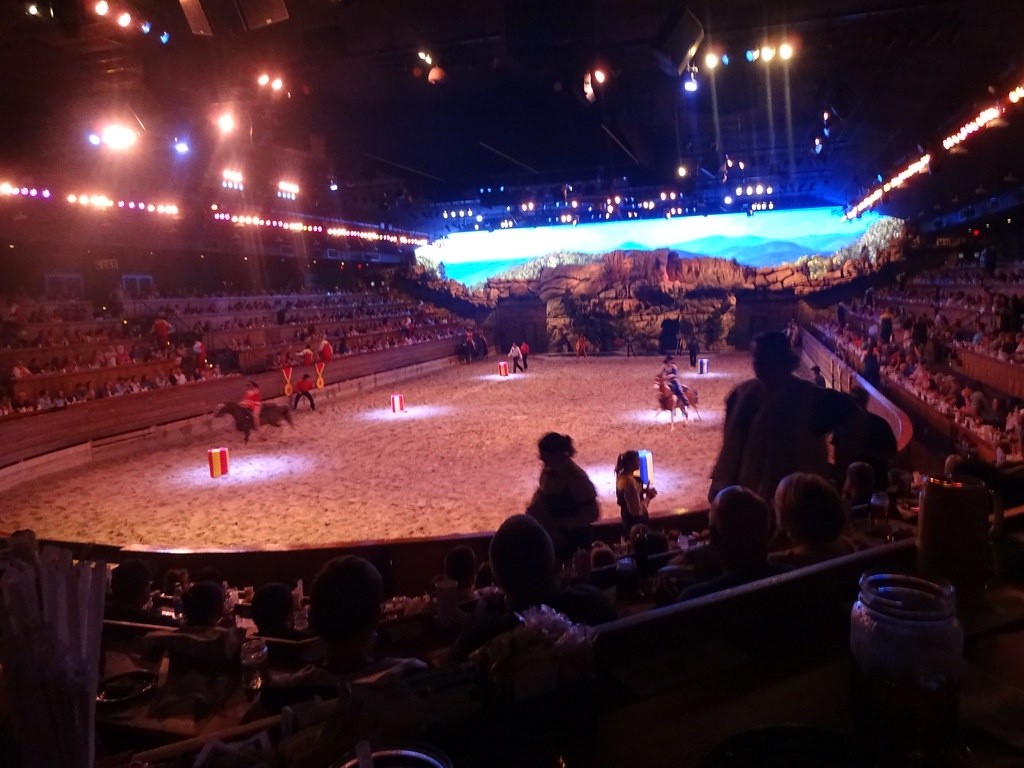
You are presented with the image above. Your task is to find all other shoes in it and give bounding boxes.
[684,400,689,405]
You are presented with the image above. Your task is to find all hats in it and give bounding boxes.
[664,355,674,363]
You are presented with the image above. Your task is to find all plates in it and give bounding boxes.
[96,671,158,703]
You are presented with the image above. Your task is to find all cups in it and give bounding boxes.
[329,741,453,768]
[241,640,270,689]
[850,570,962,703]
[870,491,889,522]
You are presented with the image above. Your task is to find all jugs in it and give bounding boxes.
[917,471,1004,586]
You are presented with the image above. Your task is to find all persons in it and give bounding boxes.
[626,333,638,357]
[657,354,690,408]
[507,341,524,372]
[293,373,316,412]
[520,340,530,368]
[687,337,700,367]
[614,449,657,523]
[676,329,687,356]
[238,381,263,432]
[0,225,1024,768]
[576,332,588,359]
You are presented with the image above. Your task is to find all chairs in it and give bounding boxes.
[0,258,1024,768]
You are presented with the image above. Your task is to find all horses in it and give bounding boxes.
[214,402,297,445]
[651,372,701,432]
[460,334,488,365]
